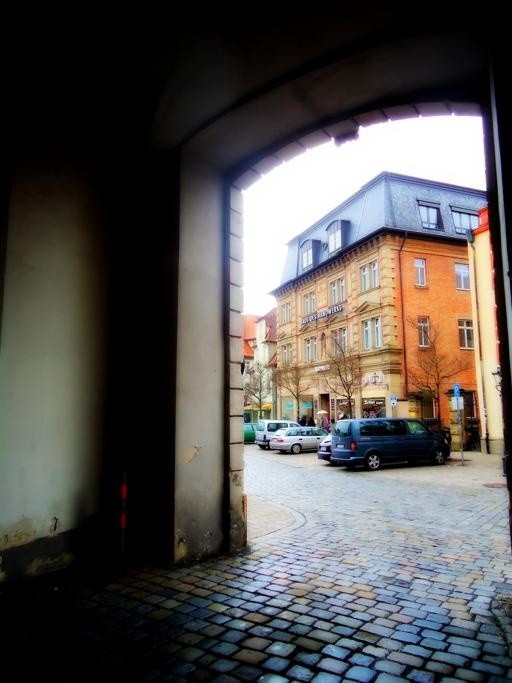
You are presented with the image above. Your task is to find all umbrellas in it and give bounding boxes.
[316,408,328,414]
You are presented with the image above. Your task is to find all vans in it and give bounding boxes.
[329,416,453,471]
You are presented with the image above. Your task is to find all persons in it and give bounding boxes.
[298,414,331,428]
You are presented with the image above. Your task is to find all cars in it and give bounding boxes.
[244,415,335,465]
